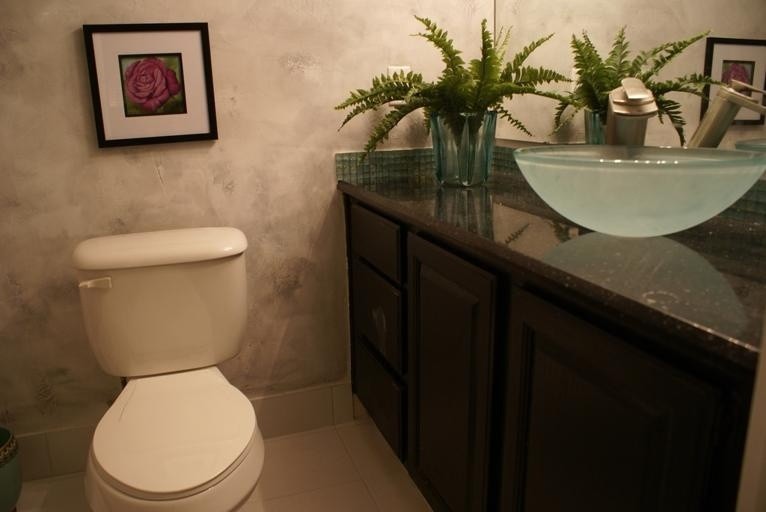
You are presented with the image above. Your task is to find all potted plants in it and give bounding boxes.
[340,16,578,191]
[555,28,732,148]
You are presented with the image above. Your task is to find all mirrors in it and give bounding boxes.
[492,0,766,155]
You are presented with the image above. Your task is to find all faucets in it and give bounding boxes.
[684,78,766,147]
[604,77,659,145]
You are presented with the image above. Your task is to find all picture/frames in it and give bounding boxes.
[80,20,222,152]
[696,33,766,129]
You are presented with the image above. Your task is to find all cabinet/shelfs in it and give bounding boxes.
[502,257,762,511]
[336,182,410,464]
[399,226,513,512]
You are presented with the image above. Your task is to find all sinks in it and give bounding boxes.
[733,132,765,151]
[515,144,765,239]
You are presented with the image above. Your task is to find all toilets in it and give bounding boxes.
[71,223,267,510]
